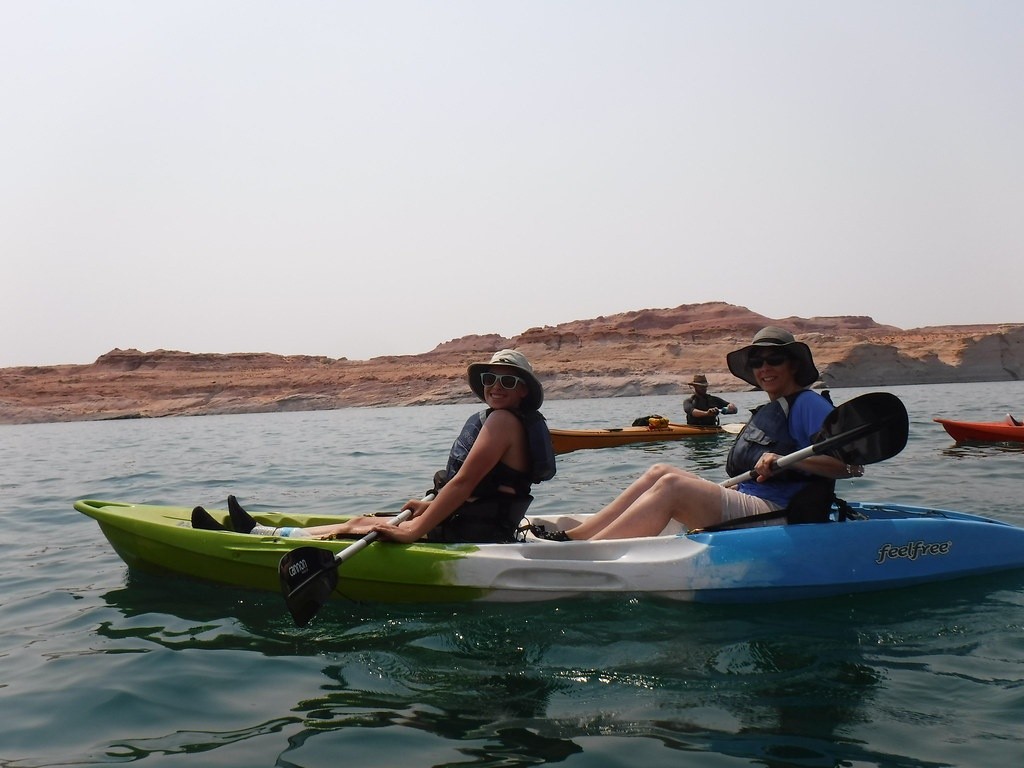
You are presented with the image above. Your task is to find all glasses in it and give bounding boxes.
[480,373,525,390]
[748,352,791,368]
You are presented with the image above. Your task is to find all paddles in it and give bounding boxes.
[276,469,447,630]
[693,423,746,434]
[718,390,910,488]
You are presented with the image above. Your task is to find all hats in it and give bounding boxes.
[688,374,712,386]
[467,349,544,410]
[726,326,819,387]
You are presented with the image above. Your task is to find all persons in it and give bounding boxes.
[531,326,866,541]
[683,374,738,427]
[1006,414,1024,426]
[191,349,557,543]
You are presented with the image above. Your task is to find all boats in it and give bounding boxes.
[71,495,1023,606]
[931,416,1024,443]
[546,422,727,454]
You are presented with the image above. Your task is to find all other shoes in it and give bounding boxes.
[228,495,256,533]
[531,523,570,541]
[1006,413,1022,426]
[191,506,226,530]
